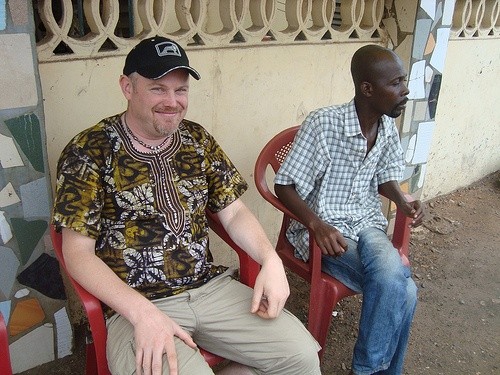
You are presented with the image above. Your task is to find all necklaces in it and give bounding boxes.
[124,110,172,151]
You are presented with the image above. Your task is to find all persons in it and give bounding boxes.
[272,45,427,375]
[51,34,324,375]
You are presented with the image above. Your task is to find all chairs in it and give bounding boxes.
[49,205,260,375]
[254,126,414,367]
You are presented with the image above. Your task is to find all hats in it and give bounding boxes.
[123,37,201,80]
